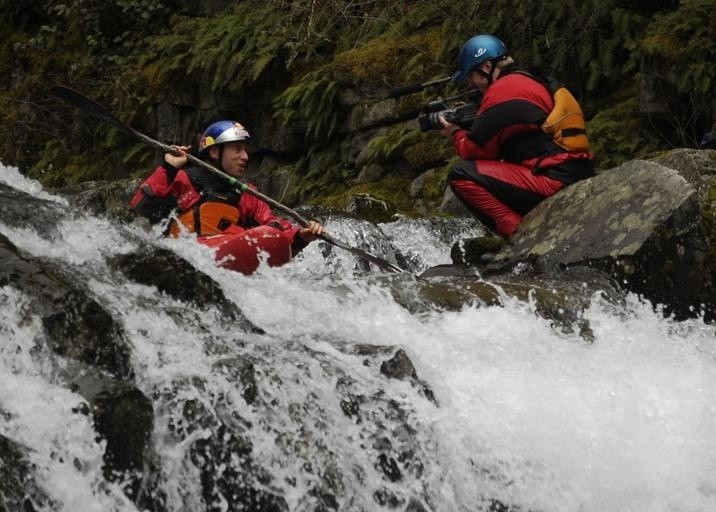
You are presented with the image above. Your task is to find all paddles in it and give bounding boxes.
[52,80,429,284]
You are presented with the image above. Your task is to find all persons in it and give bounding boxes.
[129,121,326,258]
[437,35,596,261]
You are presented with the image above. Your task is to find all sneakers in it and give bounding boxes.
[480,252,496,265]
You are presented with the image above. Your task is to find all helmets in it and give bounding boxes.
[452,34,508,82]
[198,119,250,153]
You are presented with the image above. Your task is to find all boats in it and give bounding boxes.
[215,220,292,275]
